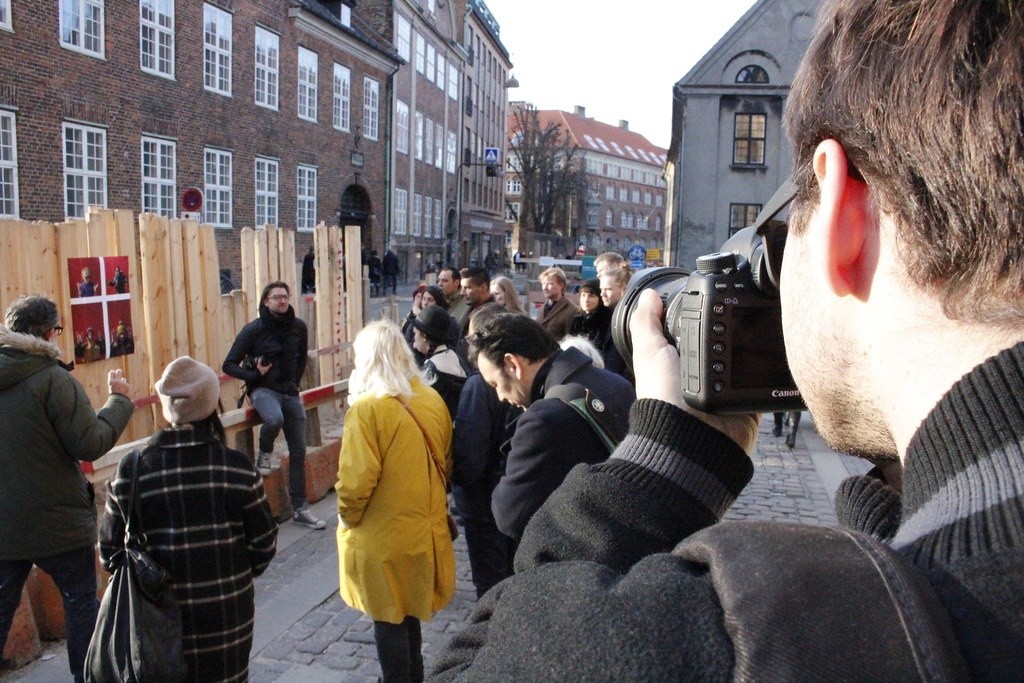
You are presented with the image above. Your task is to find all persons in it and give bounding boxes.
[107,267,129,294]
[335,320,456,683]
[463,315,637,538]
[485,248,526,273]
[97,357,279,683]
[368,249,401,297]
[223,283,325,528]
[76,328,105,363]
[77,268,98,297]
[112,320,134,356]
[422,0,1024,682]
[405,251,631,592]
[1,294,135,682]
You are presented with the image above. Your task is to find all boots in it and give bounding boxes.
[772,413,783,436]
[786,419,798,447]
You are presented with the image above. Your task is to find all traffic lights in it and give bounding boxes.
[486,165,496,177]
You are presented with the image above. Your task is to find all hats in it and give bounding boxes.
[154,356,220,424]
[407,305,452,343]
[117,325,127,337]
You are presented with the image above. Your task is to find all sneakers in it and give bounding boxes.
[294,511,326,529]
[257,452,272,477]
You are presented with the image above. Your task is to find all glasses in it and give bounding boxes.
[754,158,864,290]
[268,295,290,302]
[55,325,63,335]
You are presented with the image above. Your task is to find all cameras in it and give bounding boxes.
[242,353,270,371]
[610,220,809,411]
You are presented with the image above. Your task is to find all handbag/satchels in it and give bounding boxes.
[447,514,457,541]
[80,448,187,683]
